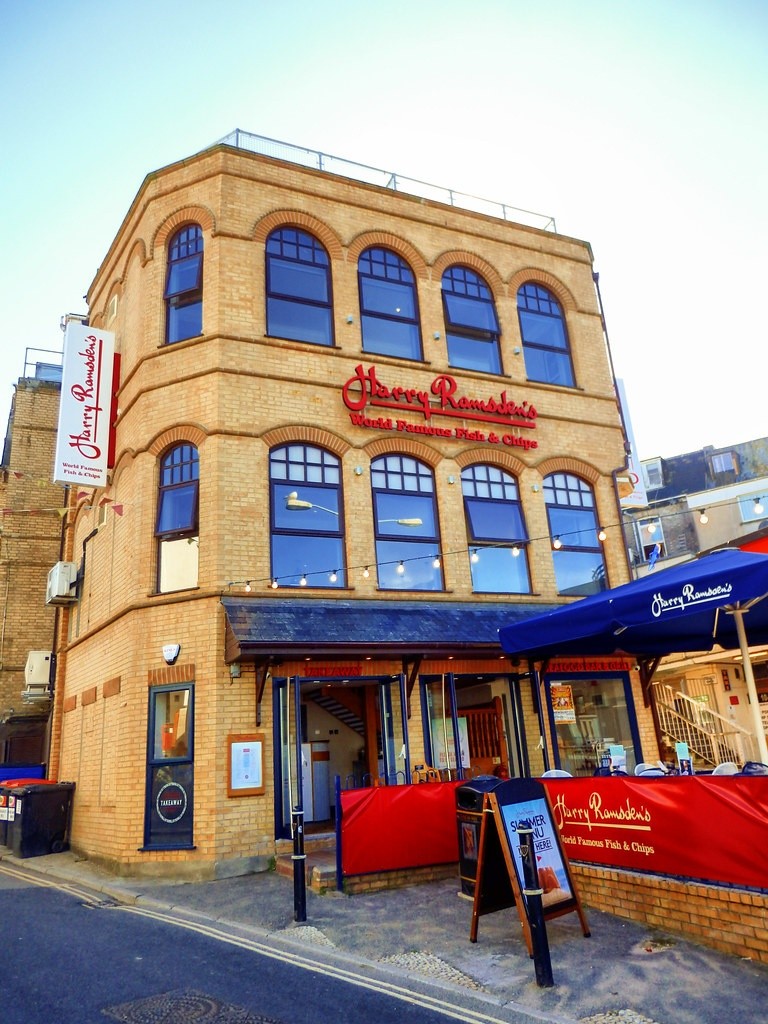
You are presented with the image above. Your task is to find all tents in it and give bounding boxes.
[497,542,768,773]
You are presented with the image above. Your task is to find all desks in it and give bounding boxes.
[664,769,714,775]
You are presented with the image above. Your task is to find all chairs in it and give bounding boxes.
[541,762,741,777]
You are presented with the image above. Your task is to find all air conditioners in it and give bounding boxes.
[45,561,77,606]
[25,651,51,695]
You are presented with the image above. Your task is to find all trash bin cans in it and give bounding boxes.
[0,778,43,846]
[455,775,503,902]
[12,780,76,859]
[5,778,56,850]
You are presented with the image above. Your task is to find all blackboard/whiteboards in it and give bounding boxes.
[488,777,578,921]
